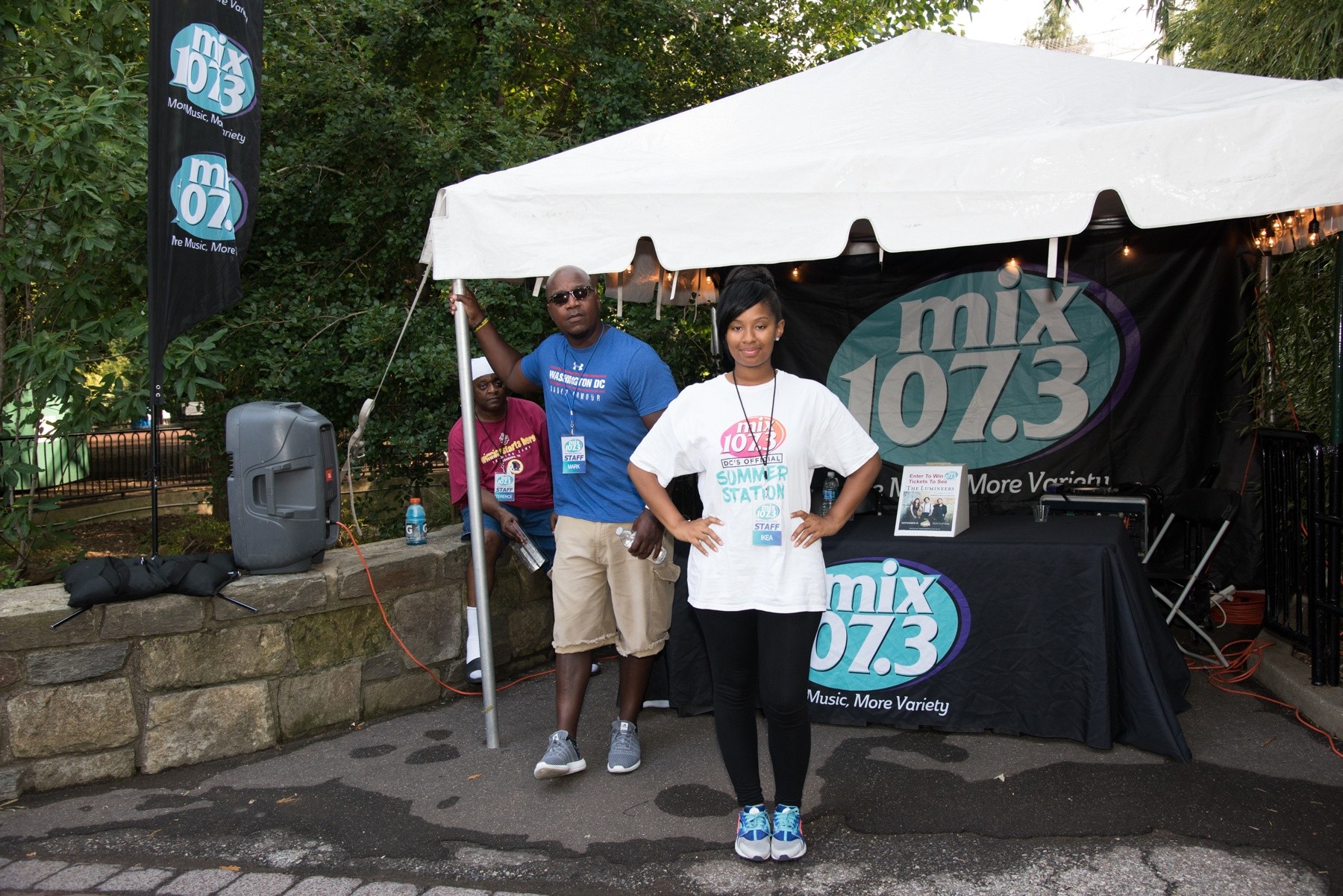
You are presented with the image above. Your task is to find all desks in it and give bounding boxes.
[615,515,1191,768]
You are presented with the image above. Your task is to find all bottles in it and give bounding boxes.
[615,526,667,564]
[405,498,427,545]
[822,472,838,517]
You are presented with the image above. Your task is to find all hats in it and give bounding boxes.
[471,356,495,381]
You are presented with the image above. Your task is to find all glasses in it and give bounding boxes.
[548,287,595,307]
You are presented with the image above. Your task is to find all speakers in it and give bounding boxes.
[225,401,340,576]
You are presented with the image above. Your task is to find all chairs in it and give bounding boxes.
[1138,487,1242,669]
[811,483,884,516]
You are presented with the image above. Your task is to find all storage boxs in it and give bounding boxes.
[1039,485,1152,564]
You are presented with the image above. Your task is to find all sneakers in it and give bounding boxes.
[735,804,771,861]
[606,719,641,773]
[770,804,807,861]
[534,730,587,780]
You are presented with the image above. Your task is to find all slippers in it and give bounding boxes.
[465,658,482,683]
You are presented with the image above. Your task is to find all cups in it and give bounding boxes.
[1032,505,1050,523]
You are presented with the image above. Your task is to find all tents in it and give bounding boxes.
[420,27,1343,755]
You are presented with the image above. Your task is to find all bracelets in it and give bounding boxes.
[645,504,650,509]
[469,315,489,333]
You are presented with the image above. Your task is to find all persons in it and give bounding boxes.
[626,266,882,869]
[899,498,947,531]
[447,266,678,781]
[448,355,602,683]
